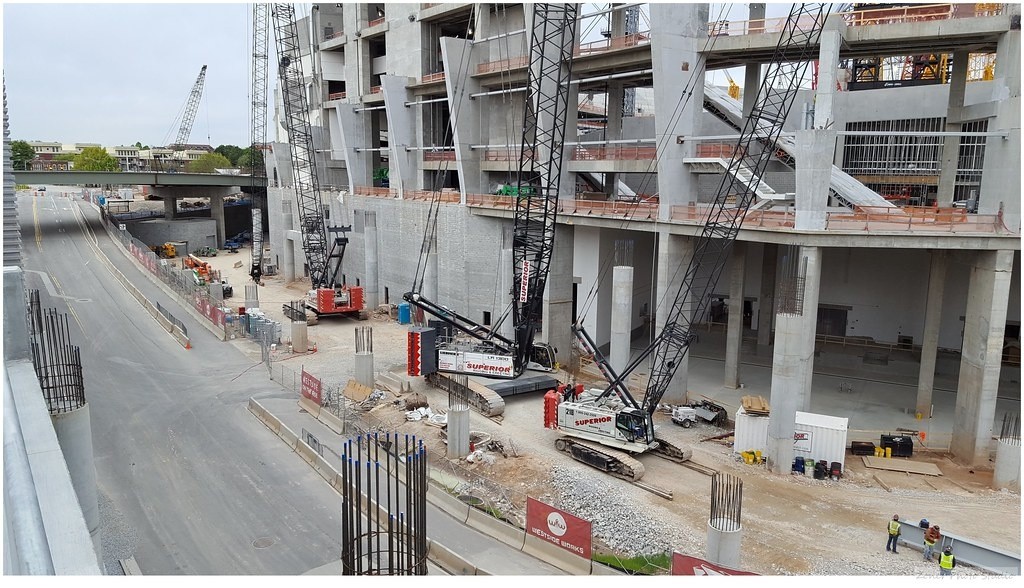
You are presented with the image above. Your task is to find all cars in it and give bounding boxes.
[179,193,245,209]
[37,185,47,190]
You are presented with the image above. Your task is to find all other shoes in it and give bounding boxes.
[888,548,891,551]
[892,551,899,554]
[923,558,927,563]
[927,558,933,561]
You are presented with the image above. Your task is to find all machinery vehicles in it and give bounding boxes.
[543,4,833,503]
[400,2,571,428]
[272,3,368,326]
[179,252,212,284]
[143,64,209,201]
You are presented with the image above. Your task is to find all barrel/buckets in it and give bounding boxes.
[794,456,841,481]
[226,306,259,334]
[874,445,892,458]
[741,450,762,465]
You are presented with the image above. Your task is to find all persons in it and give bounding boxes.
[562,383,576,404]
[921,524,944,566]
[884,513,903,554]
[629,417,644,439]
[937,544,958,577]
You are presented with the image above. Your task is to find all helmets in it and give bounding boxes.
[892,514,899,520]
[932,525,940,531]
[944,545,953,552]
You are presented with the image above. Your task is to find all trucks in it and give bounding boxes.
[146,243,175,260]
